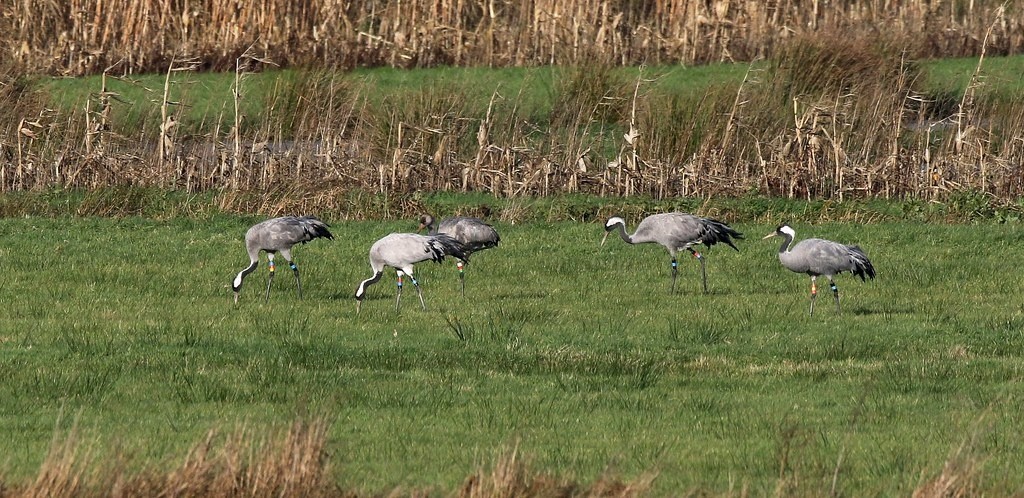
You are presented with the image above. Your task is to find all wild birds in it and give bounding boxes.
[416,213,502,303]
[353,231,475,317]
[232,212,336,310]
[600,211,746,297]
[761,224,878,318]
